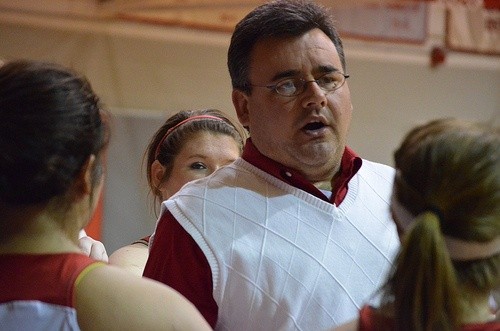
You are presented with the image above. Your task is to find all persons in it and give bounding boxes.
[108,111,243,278]
[0,59,214,331]
[322,116,500,331]
[142,0,403,331]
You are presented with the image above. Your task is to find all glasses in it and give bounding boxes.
[240,72,349,97]
